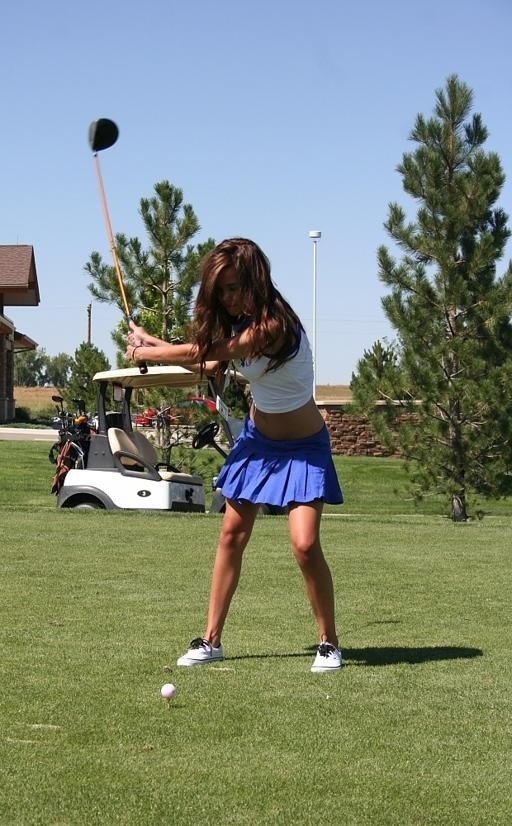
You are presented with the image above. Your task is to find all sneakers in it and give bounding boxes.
[309,632,342,673]
[176,637,223,666]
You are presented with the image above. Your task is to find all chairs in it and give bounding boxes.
[106,428,205,485]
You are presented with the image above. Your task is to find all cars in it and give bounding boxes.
[132,395,219,426]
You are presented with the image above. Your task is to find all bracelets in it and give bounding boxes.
[132,345,141,360]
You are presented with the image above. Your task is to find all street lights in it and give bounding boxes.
[306,229,323,400]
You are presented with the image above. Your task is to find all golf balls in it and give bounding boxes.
[161,684,176,698]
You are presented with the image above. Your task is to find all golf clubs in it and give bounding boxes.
[51,396,85,430]
[88,118,148,373]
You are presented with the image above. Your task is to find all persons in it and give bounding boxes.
[125,237,343,674]
